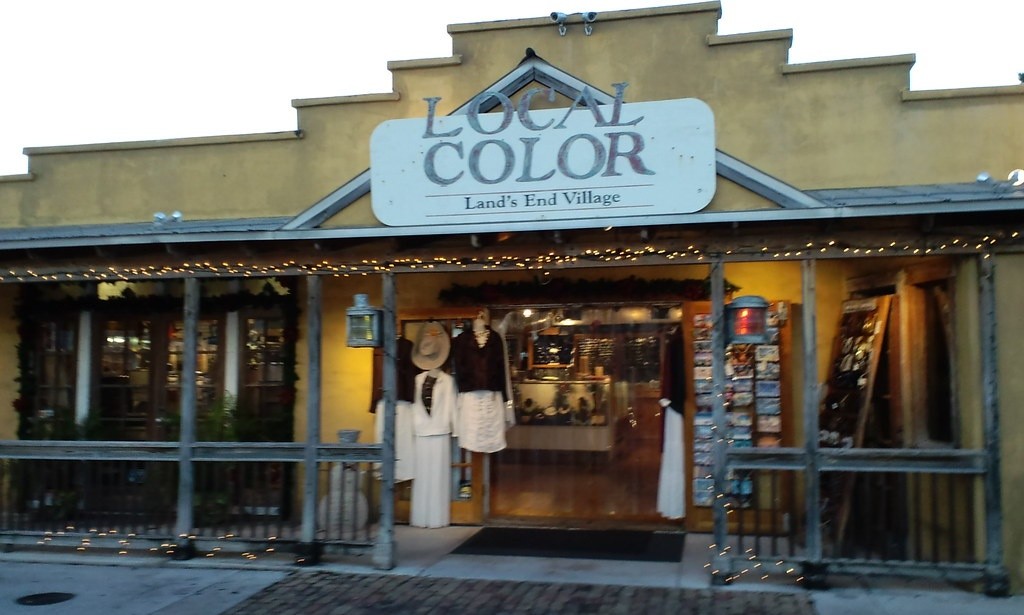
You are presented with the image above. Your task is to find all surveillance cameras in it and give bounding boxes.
[581,12,597,22]
[550,12,566,23]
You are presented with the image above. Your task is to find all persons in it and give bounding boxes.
[449,318,508,454]
[409,368,458,528]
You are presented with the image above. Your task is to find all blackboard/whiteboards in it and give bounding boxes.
[817,293,893,515]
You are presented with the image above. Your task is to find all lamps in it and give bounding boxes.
[345,293,383,348]
[581,12,597,36]
[549,11,567,35]
[725,295,769,348]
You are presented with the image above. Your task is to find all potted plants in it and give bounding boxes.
[137,387,255,529]
[0,406,105,531]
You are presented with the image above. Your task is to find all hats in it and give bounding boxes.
[411,321,450,369]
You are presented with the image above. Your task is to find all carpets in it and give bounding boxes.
[450,526,688,563]
